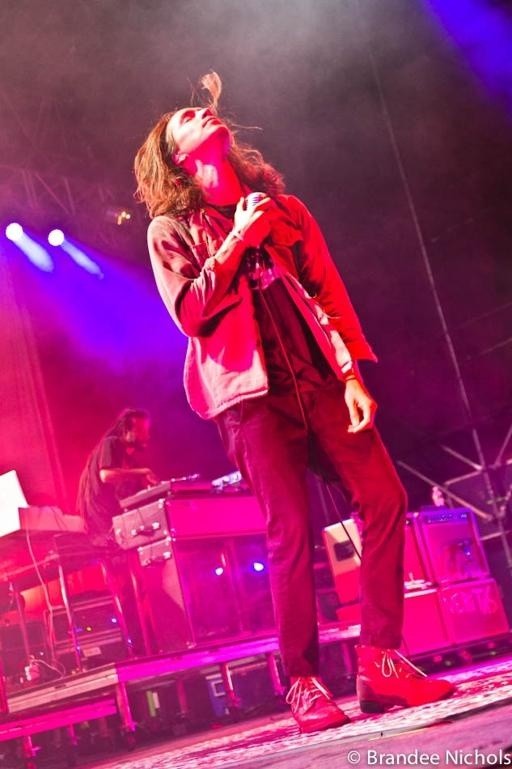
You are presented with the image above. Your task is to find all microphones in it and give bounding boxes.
[245,190,269,267]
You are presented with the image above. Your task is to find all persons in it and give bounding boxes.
[134,74,461,738]
[75,404,163,657]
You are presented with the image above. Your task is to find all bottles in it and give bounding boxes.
[432,486,445,506]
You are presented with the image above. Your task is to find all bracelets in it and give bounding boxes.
[228,228,252,250]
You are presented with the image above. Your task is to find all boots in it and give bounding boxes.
[353,644,455,713]
[285,675,350,732]
